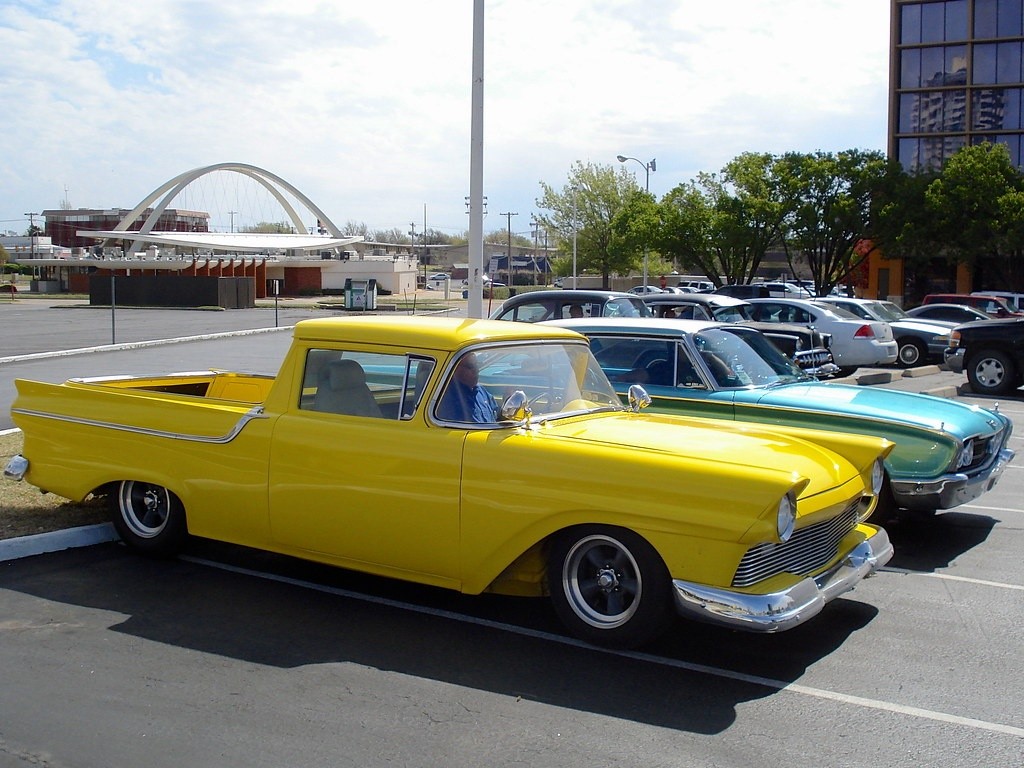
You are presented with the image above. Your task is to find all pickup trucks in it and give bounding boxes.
[3,316,896,639]
[344,317,1016,529]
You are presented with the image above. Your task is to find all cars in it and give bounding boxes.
[712,297,899,378]
[772,291,1024,368]
[554,278,565,288]
[461,283,506,299]
[487,290,843,385]
[430,273,450,280]
[944,315,1024,395]
[0,285,17,292]
[417,275,434,290]
[625,278,855,315]
[462,275,492,284]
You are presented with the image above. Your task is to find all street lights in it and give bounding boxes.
[571,182,588,289]
[228,210,237,233]
[617,154,656,295]
[25,213,38,279]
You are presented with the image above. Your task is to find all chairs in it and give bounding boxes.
[413,358,434,410]
[312,358,384,418]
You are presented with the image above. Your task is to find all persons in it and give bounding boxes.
[437,353,501,424]
[568,304,584,318]
[615,338,736,387]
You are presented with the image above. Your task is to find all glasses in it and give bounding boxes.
[458,363,477,369]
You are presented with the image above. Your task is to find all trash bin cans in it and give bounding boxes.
[217,276,237,309]
[237,276,257,309]
[343,277,377,311]
